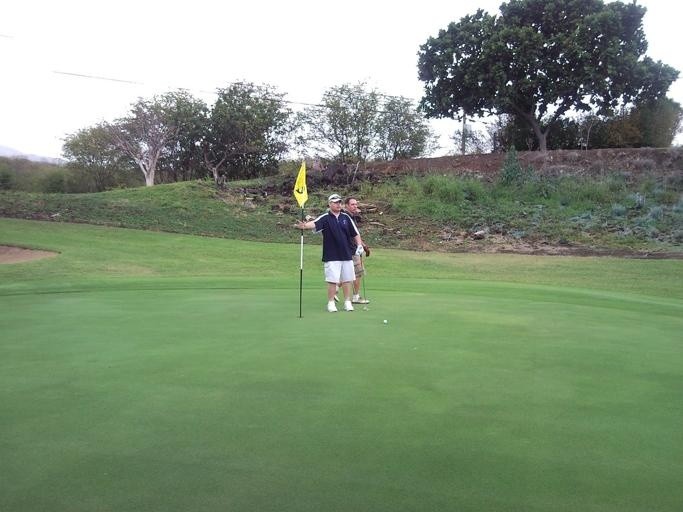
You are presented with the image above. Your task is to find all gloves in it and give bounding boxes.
[364,245,370,257]
[355,245,365,256]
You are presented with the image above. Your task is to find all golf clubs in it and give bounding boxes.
[359,246,368,311]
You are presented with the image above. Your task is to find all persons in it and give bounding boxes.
[342,196,370,305]
[293,193,363,312]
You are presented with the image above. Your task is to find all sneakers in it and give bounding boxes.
[351,296,371,304]
[343,300,354,311]
[327,301,338,312]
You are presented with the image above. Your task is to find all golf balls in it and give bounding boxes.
[383,320,387,323]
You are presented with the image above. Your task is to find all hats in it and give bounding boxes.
[328,193,343,203]
